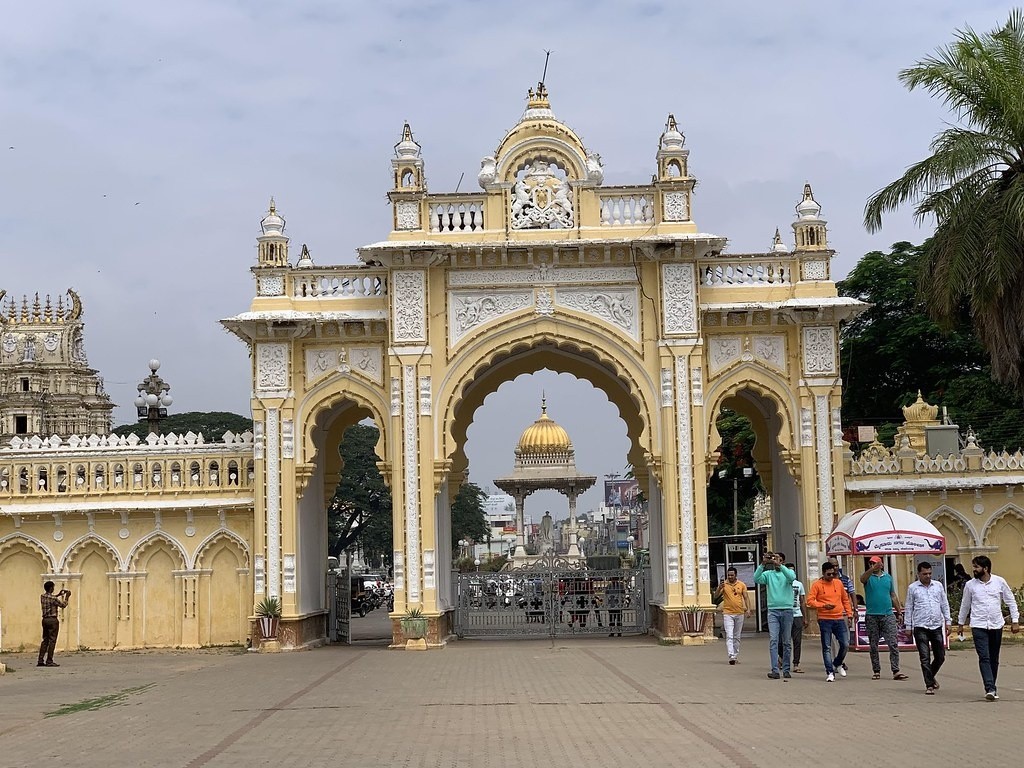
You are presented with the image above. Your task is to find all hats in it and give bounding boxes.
[868,556,881,563]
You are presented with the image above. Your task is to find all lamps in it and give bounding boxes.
[772,227,788,253]
[297,244,314,270]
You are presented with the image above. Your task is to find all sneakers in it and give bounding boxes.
[985,690,999,700]
[837,666,846,676]
[826,672,836,682]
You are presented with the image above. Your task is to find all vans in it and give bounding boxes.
[351,574,393,597]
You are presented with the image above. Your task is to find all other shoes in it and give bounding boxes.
[38,662,46,666]
[784,671,791,678]
[47,663,57,666]
[768,672,780,678]
[729,658,739,664]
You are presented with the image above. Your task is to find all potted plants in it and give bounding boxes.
[255,596,284,638]
[402,608,430,640]
[678,605,709,633]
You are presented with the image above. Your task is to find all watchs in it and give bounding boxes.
[898,613,904,617]
[854,609,858,612]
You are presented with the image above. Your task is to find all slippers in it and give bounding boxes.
[793,668,804,673]
[872,674,880,679]
[925,689,935,695]
[933,681,940,689]
[778,660,783,669]
[893,674,908,680]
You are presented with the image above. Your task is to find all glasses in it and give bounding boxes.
[832,565,838,568]
[733,589,737,595]
[824,572,835,576]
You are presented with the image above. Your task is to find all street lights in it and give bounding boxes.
[627,536,636,558]
[463,541,469,559]
[474,560,481,572]
[459,540,464,558]
[506,538,514,561]
[718,467,756,539]
[499,531,505,556]
[133,356,173,435]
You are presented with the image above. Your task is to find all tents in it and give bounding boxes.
[826,503,950,648]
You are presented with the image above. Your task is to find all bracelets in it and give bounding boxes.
[848,617,853,620]
[719,586,722,589]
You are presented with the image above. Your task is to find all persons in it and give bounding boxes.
[957,556,1020,700]
[558,566,603,628]
[609,489,623,506]
[828,556,859,672]
[714,567,750,664]
[752,551,797,679]
[954,564,972,588]
[905,561,953,695]
[541,511,552,539]
[777,562,809,673]
[860,555,909,680]
[806,561,853,681]
[605,573,625,637]
[36,581,71,667]
